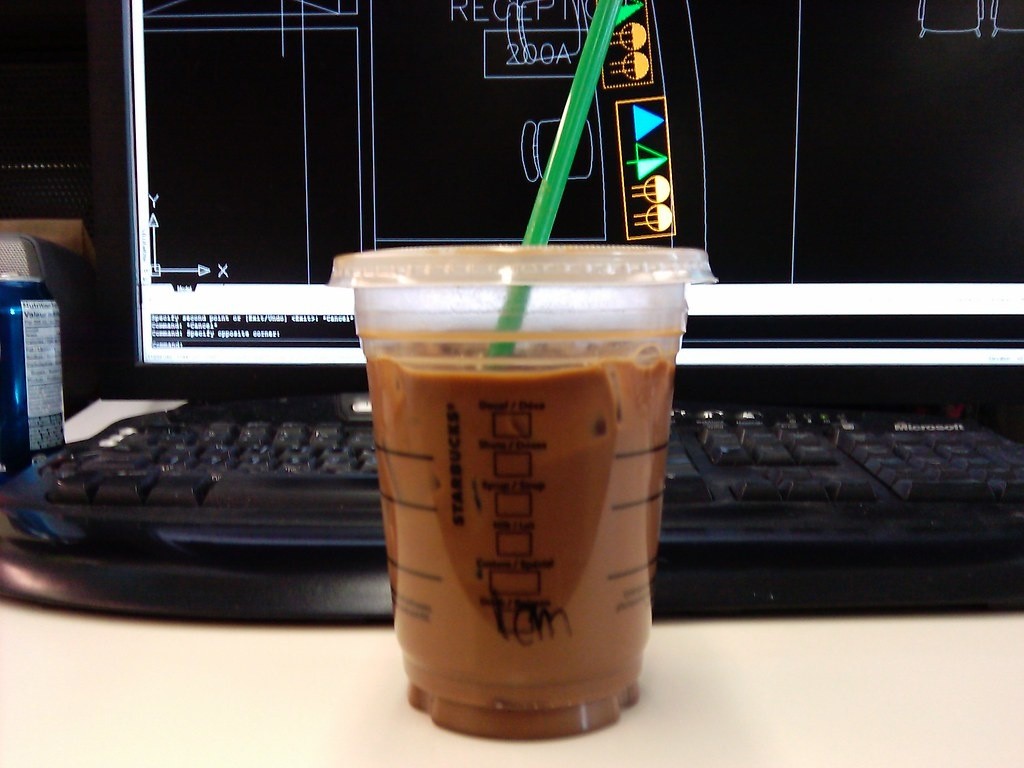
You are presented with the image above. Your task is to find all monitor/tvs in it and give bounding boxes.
[86,0,1024,405]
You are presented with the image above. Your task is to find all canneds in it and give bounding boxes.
[0,272,66,482]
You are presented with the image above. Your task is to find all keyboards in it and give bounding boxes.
[0,399,1024,623]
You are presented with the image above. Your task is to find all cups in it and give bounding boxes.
[330,242,711,742]
